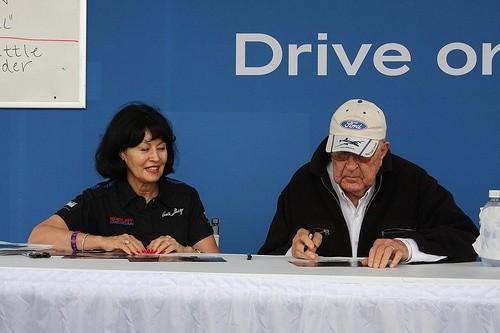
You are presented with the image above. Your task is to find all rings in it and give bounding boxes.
[123,239,130,244]
[389,245,395,252]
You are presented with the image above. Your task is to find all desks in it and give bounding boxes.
[0,253,500,332]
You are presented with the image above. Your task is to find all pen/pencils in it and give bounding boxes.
[302,228,314,252]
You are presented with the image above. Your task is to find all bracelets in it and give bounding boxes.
[191,247,201,253]
[82,233,90,252]
[71,231,80,251]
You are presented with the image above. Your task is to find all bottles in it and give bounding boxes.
[479,190,500,267]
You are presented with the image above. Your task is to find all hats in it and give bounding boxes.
[326,98,387,158]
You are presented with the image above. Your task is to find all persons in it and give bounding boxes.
[28,103,221,254]
[257,99,480,268]
